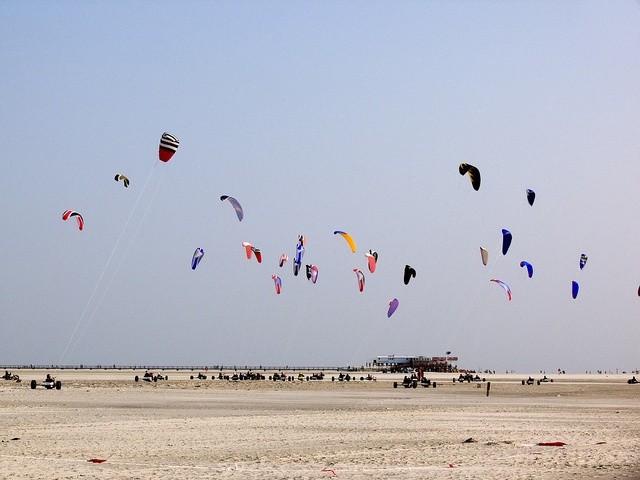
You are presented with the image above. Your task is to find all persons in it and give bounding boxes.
[46,374,55,382]
[404,376,411,383]
[455,370,483,385]
[144,371,149,377]
[4,371,9,377]
[522,373,554,387]
[411,371,432,387]
[189,369,381,383]
